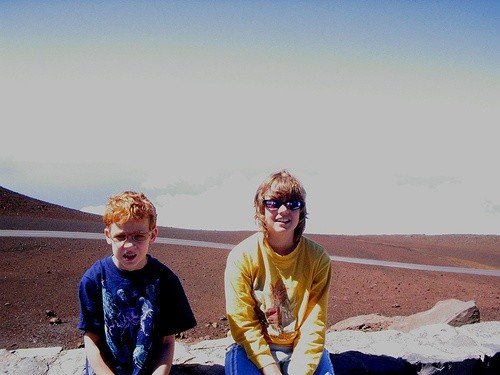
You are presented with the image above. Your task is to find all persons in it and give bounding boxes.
[76,190,197,375]
[220,170,336,375]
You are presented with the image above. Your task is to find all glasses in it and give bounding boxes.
[262,199,305,211]
[108,230,153,243]
